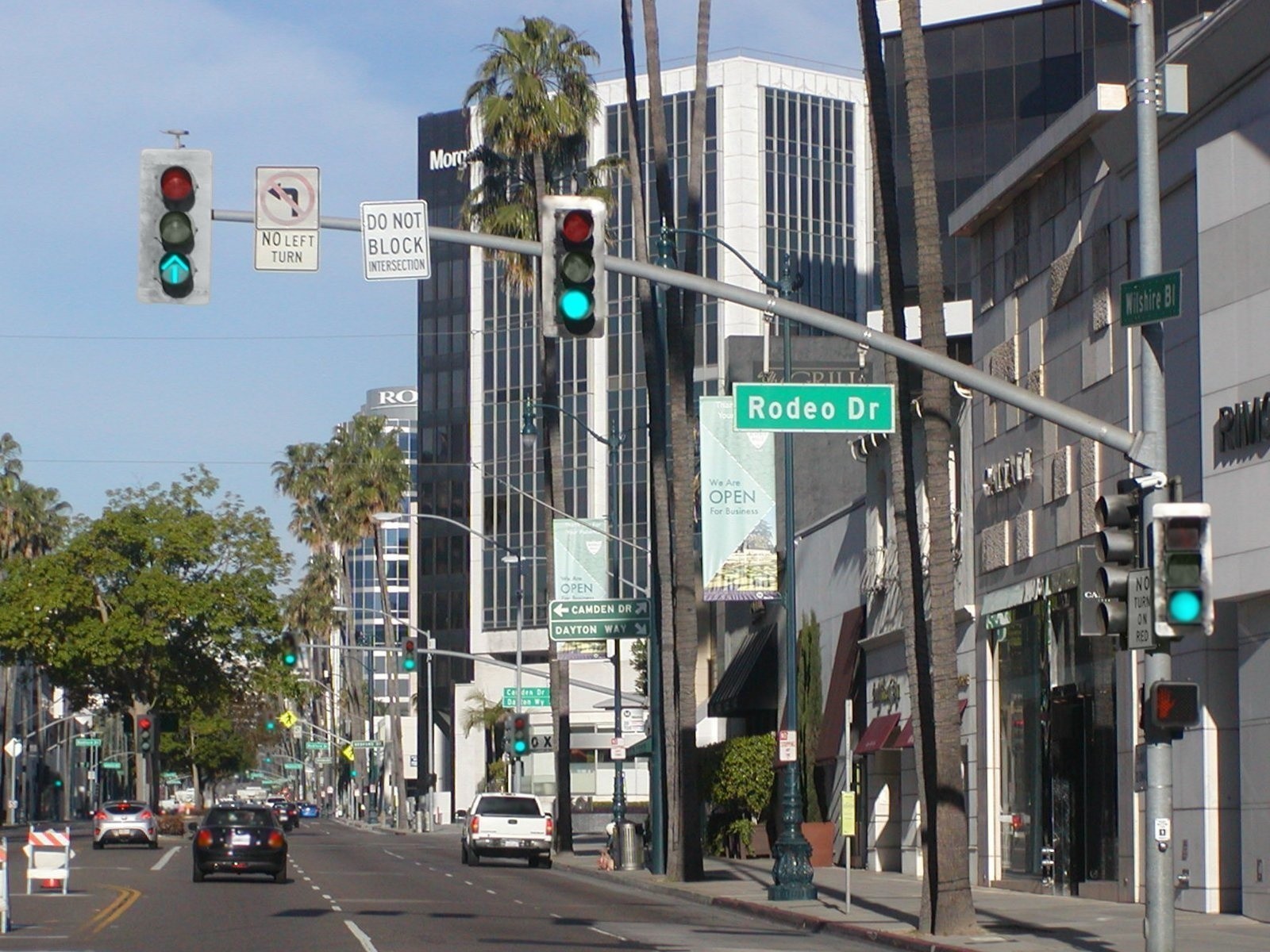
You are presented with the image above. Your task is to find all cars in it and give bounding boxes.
[90,799,159,849]
[300,803,320,818]
[208,787,301,828]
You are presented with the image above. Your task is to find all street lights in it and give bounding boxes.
[639,220,817,909]
[520,394,666,876]
[337,653,381,825]
[498,555,651,752]
[370,509,526,791]
[297,676,358,820]
[329,605,435,832]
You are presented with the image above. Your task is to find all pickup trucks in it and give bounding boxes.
[456,792,555,869]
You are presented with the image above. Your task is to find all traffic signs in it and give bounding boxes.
[546,596,652,639]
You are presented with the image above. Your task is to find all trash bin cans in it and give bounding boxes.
[622,823,641,870]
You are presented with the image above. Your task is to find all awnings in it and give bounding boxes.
[813,602,867,767]
[892,697,967,747]
[853,714,902,753]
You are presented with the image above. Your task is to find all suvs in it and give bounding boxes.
[187,802,290,884]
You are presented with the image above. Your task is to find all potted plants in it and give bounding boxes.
[698,609,834,870]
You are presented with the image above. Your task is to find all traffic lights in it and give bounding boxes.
[282,632,299,668]
[265,750,272,765]
[1090,479,1147,646]
[502,715,514,755]
[266,712,277,737]
[511,713,530,757]
[348,770,357,779]
[138,145,218,306]
[136,714,156,754]
[541,196,610,339]
[401,636,418,670]
[1151,501,1216,638]
[53,779,64,793]
[1146,681,1202,733]
